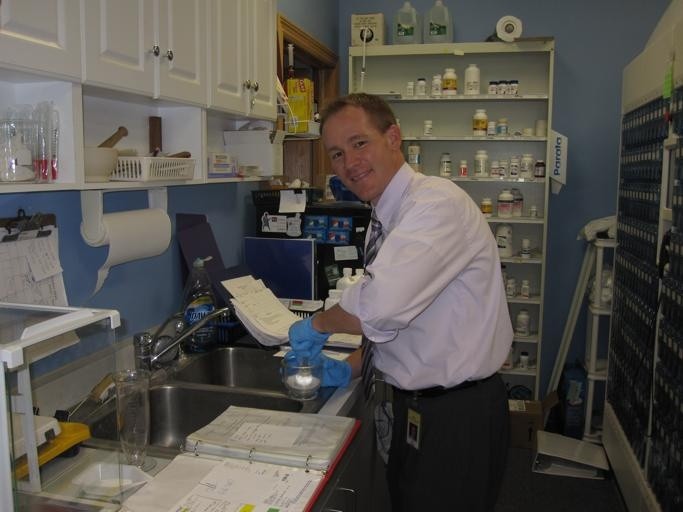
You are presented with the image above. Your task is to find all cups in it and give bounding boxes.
[113,369,157,471]
[281,355,323,402]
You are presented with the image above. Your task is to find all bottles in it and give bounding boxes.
[507,280,515,298]
[407,82,414,96]
[429,73,443,97]
[529,205,538,219]
[480,198,493,218]
[423,120,433,136]
[472,108,488,137]
[509,159,521,178]
[490,161,500,177]
[521,153,534,180]
[415,77,426,95]
[500,160,508,177]
[509,80,519,94]
[496,222,512,258]
[439,153,452,177]
[534,160,545,177]
[458,160,468,177]
[516,308,533,338]
[498,80,509,94]
[521,279,532,299]
[519,351,529,370]
[464,62,480,94]
[513,189,523,216]
[497,189,513,218]
[502,349,514,370]
[535,120,546,137]
[407,141,424,174]
[521,238,531,258]
[488,81,497,94]
[443,68,458,96]
[488,121,495,136]
[496,119,510,135]
[473,149,489,178]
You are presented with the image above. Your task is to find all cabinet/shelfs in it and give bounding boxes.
[544,236,616,445]
[347,37,556,401]
[0,0,286,194]
[1,302,394,512]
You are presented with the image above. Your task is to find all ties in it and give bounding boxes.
[361,211,384,407]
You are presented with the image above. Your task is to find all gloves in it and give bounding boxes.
[279,351,352,388]
[289,312,333,362]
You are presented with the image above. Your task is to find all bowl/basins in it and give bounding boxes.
[84,146,118,183]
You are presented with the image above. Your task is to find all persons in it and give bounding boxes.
[288,92,514,511]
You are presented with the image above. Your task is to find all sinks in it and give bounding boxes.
[67,386,333,459]
[160,343,341,393]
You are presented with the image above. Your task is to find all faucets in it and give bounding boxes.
[133,307,230,369]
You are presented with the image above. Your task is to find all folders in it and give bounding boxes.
[532,430,610,481]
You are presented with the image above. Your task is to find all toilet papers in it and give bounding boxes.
[82,209,171,298]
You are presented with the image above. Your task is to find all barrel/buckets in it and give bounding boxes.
[395,1,421,44]
[421,0,453,44]
